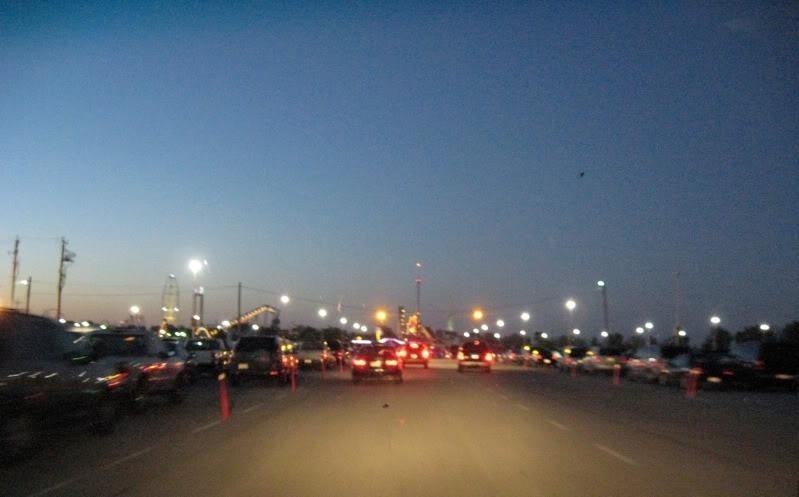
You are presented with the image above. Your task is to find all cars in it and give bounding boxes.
[1,305,234,464]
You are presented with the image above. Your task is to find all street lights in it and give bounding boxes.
[462,280,609,344]
[280,295,368,336]
